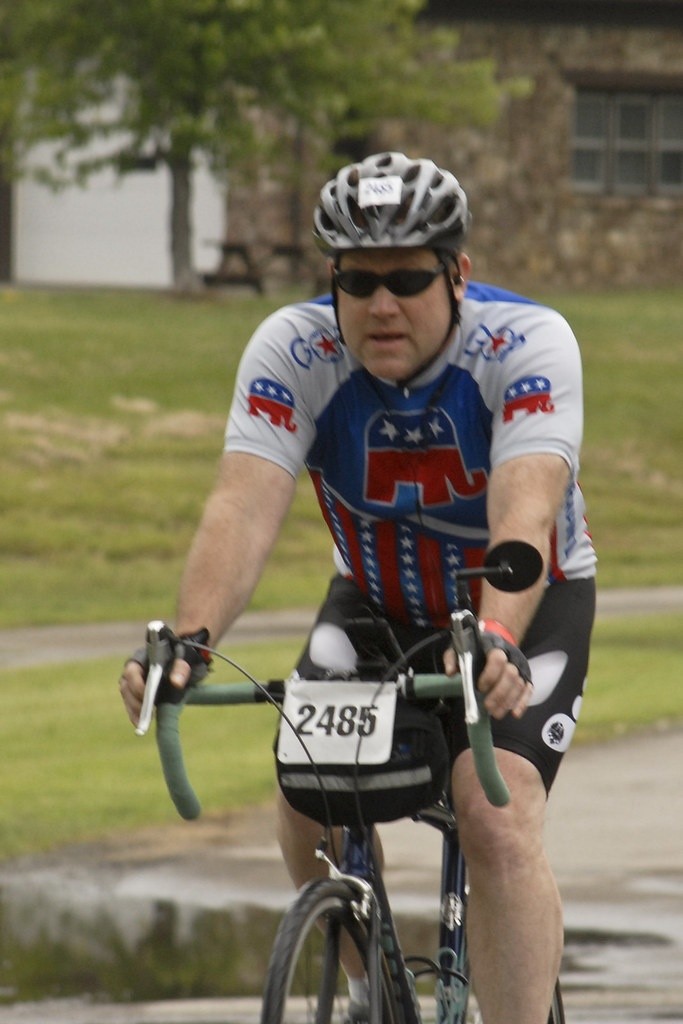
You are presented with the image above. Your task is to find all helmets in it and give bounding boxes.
[310,151,474,254]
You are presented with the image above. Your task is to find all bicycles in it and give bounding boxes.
[129,537,575,1024]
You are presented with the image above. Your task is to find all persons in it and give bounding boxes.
[120,151,598,1023]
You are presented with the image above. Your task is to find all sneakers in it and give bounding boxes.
[339,967,422,1024]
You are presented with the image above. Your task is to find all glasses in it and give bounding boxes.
[331,264,446,299]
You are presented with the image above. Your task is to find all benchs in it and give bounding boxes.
[197,234,331,300]
